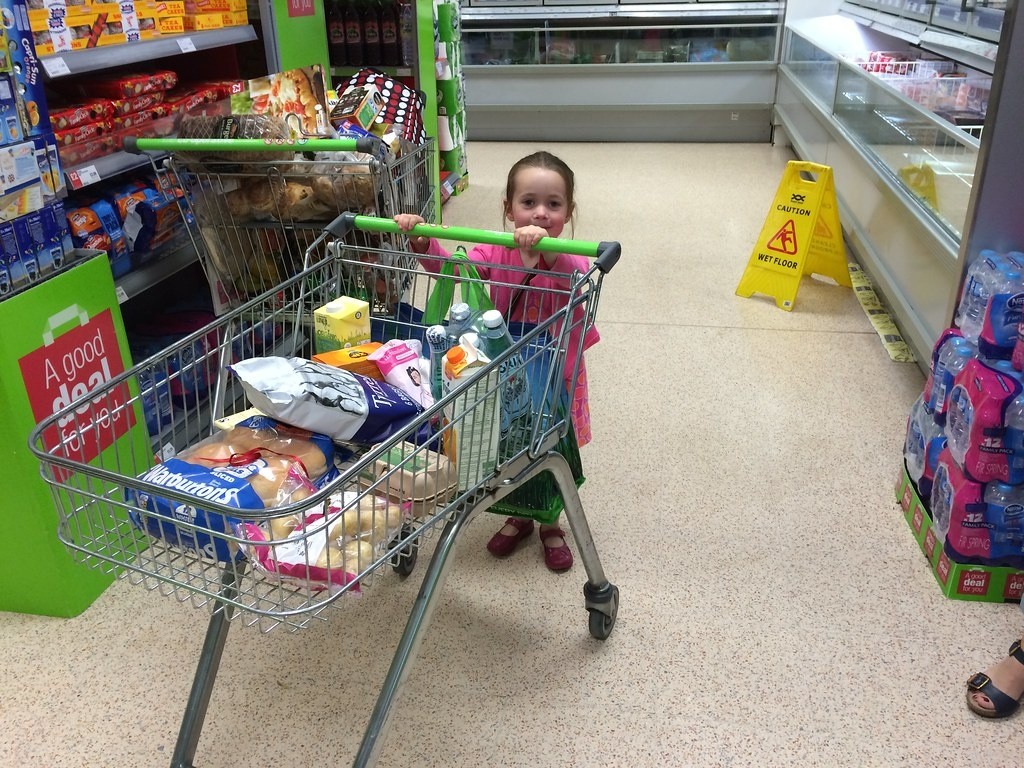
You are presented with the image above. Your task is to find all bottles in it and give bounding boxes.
[327,1,346,67]
[436,39,450,80]
[344,0,364,68]
[482,310,535,429]
[427,324,450,442]
[399,2,414,68]
[448,302,480,349]
[380,0,401,67]
[129,282,284,435]
[903,248,1024,571]
[247,243,375,300]
[437,115,453,152]
[458,330,515,453]
[360,0,381,65]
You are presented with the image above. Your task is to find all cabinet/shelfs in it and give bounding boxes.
[2,0,1024,610]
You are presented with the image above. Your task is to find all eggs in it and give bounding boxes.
[356,441,458,518]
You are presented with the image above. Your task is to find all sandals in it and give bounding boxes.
[967,640,1024,717]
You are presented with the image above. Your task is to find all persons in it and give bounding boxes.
[392,152,601,571]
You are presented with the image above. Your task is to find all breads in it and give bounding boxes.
[176,70,378,279]
[138,417,404,590]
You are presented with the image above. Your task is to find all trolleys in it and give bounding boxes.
[27,211,621,768]
[121,63,436,325]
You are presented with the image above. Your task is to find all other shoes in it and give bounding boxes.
[486,516,573,571]
